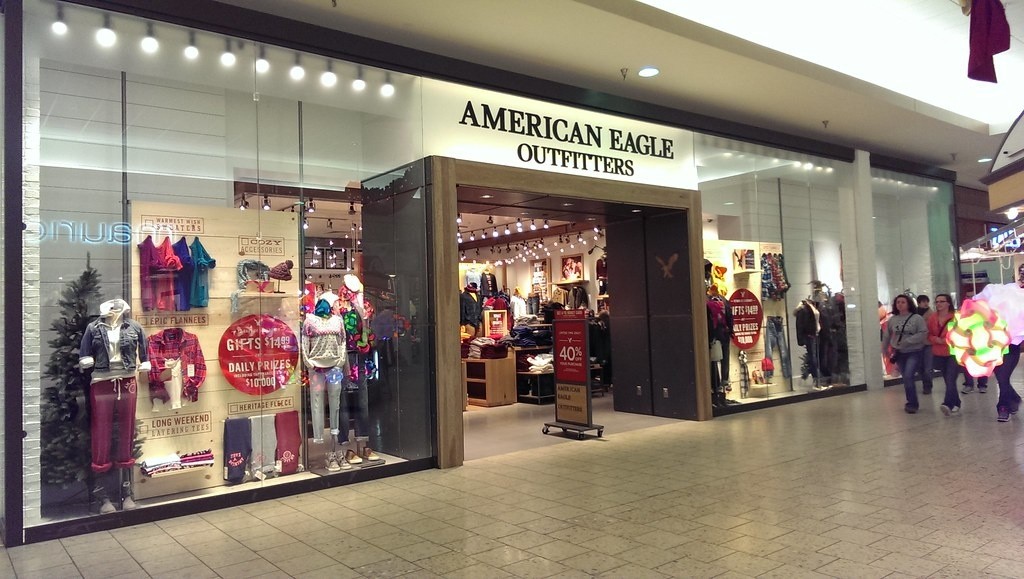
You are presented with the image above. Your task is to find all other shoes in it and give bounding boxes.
[941,402,952,416]
[905,402,917,413]
[813,383,847,391]
[952,405,962,411]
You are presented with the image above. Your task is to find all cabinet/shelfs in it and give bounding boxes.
[460,278,610,408]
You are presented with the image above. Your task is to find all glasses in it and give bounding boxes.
[934,300,948,304]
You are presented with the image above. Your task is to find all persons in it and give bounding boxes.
[993,345,1022,421]
[961,368,988,394]
[927,294,961,416]
[915,295,933,394]
[706,283,733,392]
[301,274,375,444]
[599,310,613,388]
[563,258,582,279]
[793,289,847,383]
[882,294,930,413]
[463,268,541,327]
[79,299,152,472]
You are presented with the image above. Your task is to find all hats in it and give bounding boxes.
[268,260,293,281]
[761,357,774,371]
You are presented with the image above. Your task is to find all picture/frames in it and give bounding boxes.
[559,253,584,281]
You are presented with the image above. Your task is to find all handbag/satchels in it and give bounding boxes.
[709,339,724,362]
[890,351,899,364]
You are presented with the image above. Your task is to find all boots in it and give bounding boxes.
[93,487,116,514]
[122,481,136,510]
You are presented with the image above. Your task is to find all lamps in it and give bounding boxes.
[306,199,316,213]
[347,202,356,216]
[260,195,271,210]
[456,213,605,257]
[239,194,249,210]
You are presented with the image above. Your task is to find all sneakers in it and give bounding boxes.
[979,387,986,393]
[363,447,379,460]
[324,452,341,471]
[961,384,974,393]
[345,449,363,463]
[1009,396,1022,414]
[334,449,351,470]
[998,406,1010,421]
